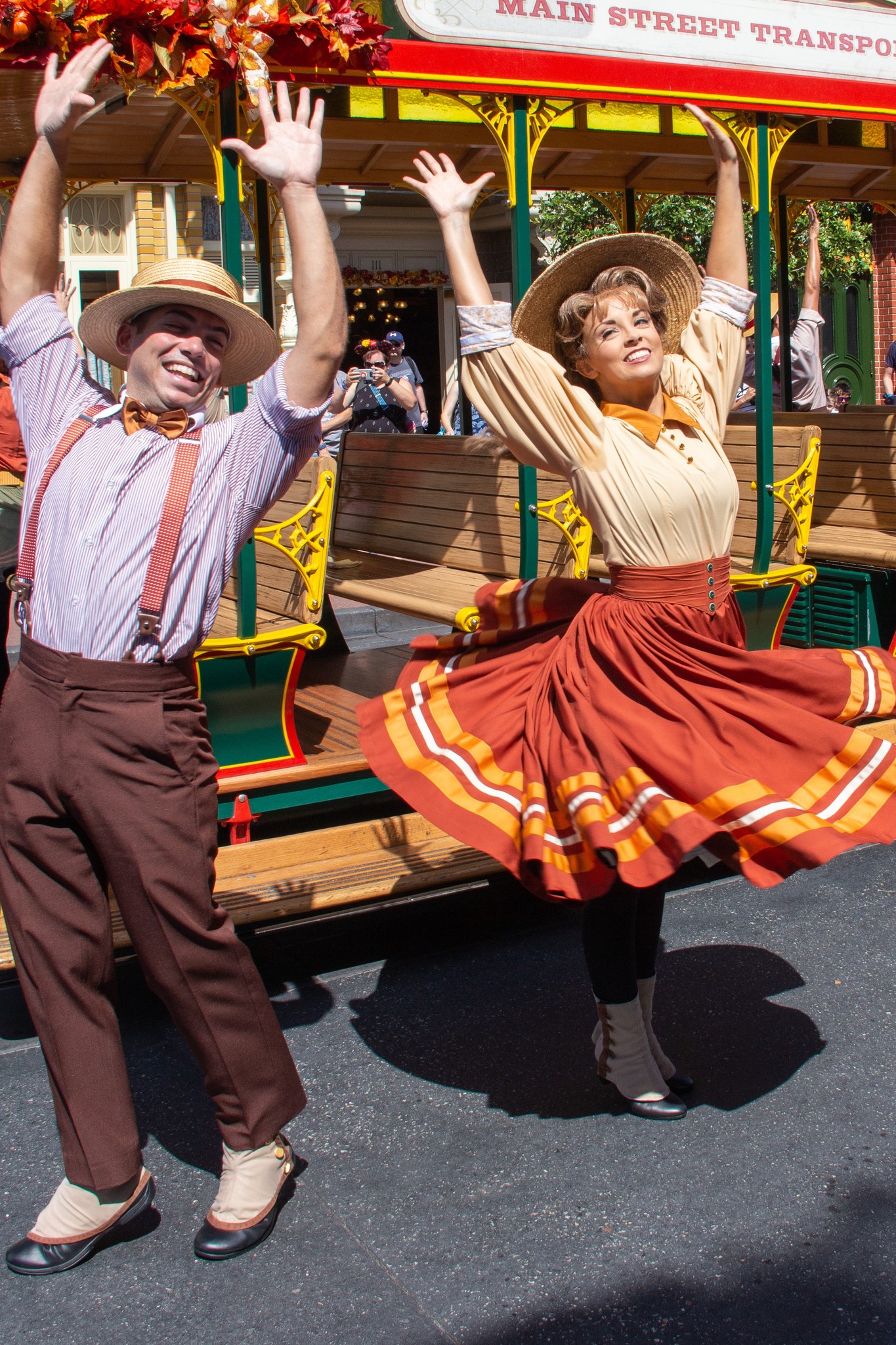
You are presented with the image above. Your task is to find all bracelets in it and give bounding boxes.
[316,442,327,451]
[340,385,348,394]
[342,380,350,391]
[385,377,393,386]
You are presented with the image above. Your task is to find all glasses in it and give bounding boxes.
[837,396,851,403]
[365,361,387,369]
[392,342,401,347]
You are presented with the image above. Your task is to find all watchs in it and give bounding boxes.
[421,410,428,415]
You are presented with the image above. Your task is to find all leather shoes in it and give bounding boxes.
[195,1140,294,1261]
[5,1173,154,1275]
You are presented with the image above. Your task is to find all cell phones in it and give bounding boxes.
[353,369,376,381]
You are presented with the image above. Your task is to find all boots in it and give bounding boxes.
[589,971,693,1091]
[591,988,689,1120]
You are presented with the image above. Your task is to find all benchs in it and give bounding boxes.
[194,406,896,653]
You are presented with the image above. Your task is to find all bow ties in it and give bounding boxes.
[122,396,188,440]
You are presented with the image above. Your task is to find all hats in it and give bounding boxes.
[510,233,701,388]
[386,331,404,344]
[742,292,778,337]
[77,257,284,387]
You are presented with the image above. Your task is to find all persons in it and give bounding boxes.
[384,331,429,435]
[882,340,896,406]
[824,383,851,413]
[0,36,373,1277]
[310,370,355,466]
[349,102,896,1116]
[440,371,491,437]
[327,338,418,434]
[728,206,826,412]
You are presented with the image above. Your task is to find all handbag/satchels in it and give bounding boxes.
[405,416,417,434]
